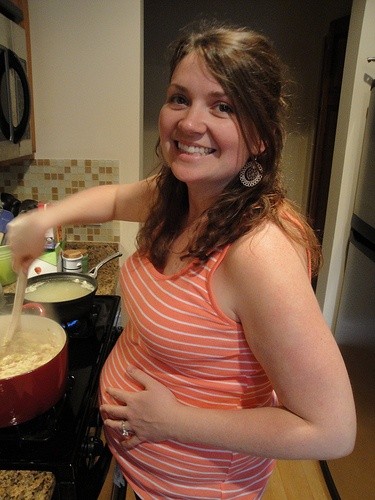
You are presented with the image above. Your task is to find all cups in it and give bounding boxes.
[0,242,18,286]
[61,253,83,274]
[82,253,88,273]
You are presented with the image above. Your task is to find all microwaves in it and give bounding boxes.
[0,14,33,161]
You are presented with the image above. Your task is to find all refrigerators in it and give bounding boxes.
[320,71,375,500]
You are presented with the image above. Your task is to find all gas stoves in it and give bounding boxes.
[0,292,121,500]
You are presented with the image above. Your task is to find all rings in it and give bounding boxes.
[121,420,131,437]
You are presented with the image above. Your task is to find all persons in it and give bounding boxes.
[5,28,359,500]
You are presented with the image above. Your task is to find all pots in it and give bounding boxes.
[0,303,69,427]
[23,251,123,323]
